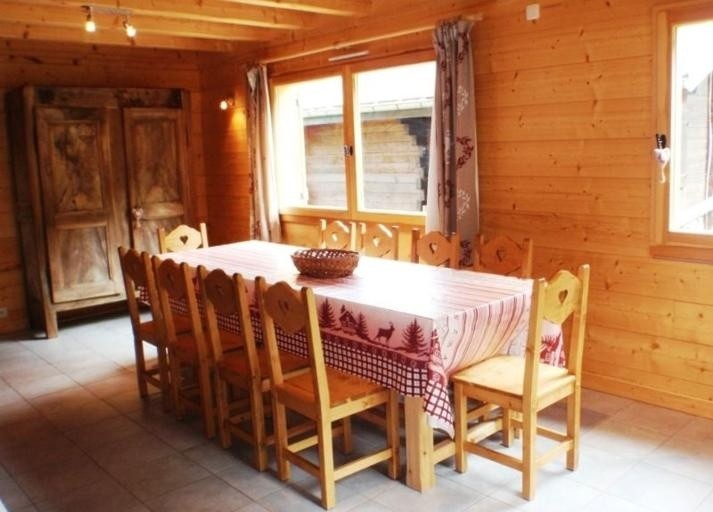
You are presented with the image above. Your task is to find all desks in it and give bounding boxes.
[155,238,570,493]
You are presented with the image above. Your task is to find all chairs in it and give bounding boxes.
[472,234,531,279]
[158,222,208,252]
[318,221,356,253]
[411,228,460,269]
[153,255,243,438]
[195,265,309,469]
[451,266,590,501]
[255,277,400,508]
[358,223,399,260]
[119,245,193,415]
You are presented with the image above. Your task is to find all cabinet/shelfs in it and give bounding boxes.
[8,83,193,338]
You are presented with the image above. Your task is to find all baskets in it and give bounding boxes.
[292,248,359,278]
